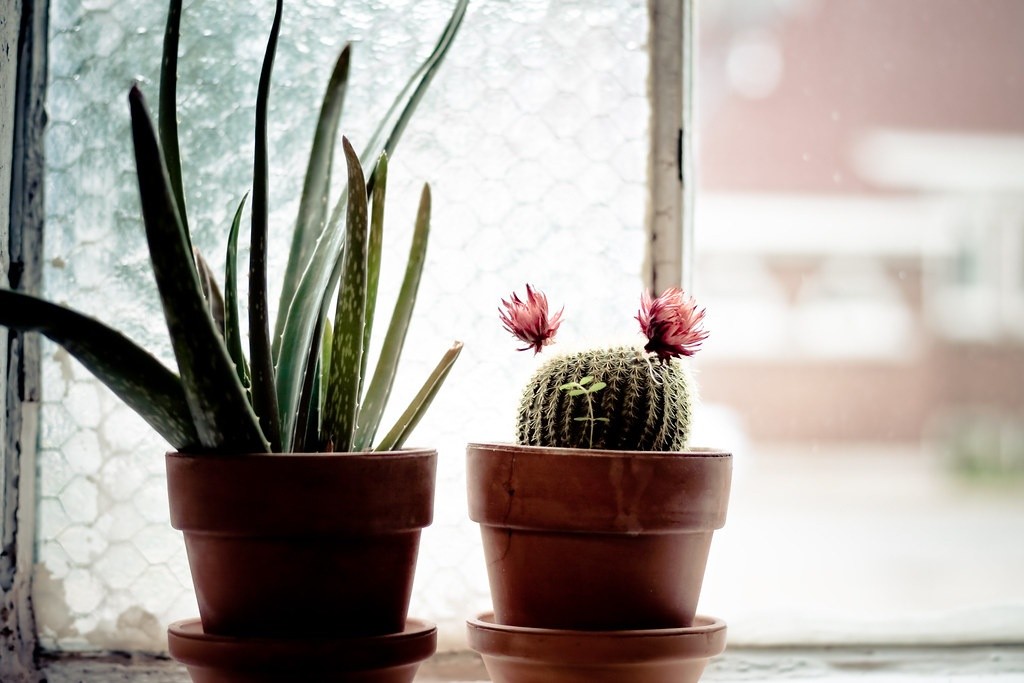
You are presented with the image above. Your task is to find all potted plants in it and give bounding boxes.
[0,0,472,683]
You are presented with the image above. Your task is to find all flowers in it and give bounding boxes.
[496,282,711,453]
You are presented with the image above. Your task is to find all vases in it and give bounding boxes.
[465,442,734,683]
